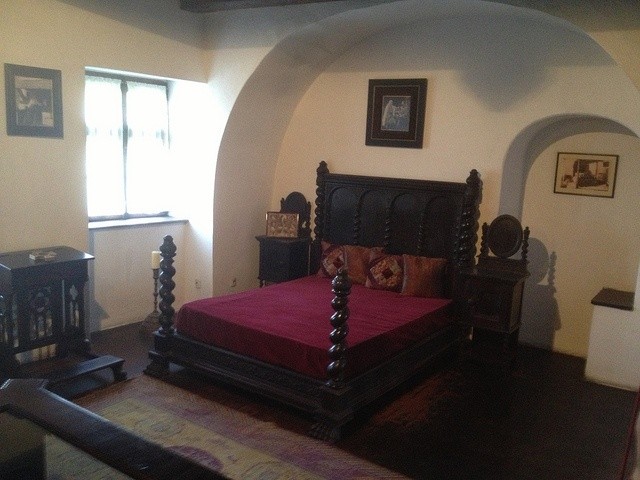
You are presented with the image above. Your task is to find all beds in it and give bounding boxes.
[145,162,483,438]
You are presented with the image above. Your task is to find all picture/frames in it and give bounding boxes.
[552,150,619,200]
[3,64,65,140]
[364,77,429,150]
[265,211,301,240]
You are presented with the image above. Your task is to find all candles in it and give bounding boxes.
[150,250,162,267]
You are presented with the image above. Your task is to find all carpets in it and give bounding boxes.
[46,375,405,479]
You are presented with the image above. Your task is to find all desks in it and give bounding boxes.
[0,247,95,368]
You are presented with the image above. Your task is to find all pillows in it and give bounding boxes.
[318,240,344,277]
[366,251,403,291]
[400,251,446,298]
[340,244,382,283]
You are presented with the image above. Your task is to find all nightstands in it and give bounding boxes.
[254,191,312,286]
[462,214,531,348]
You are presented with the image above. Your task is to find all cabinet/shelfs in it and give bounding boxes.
[585,289,639,392]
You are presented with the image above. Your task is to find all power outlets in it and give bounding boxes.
[230,276,236,286]
[195,278,201,288]
